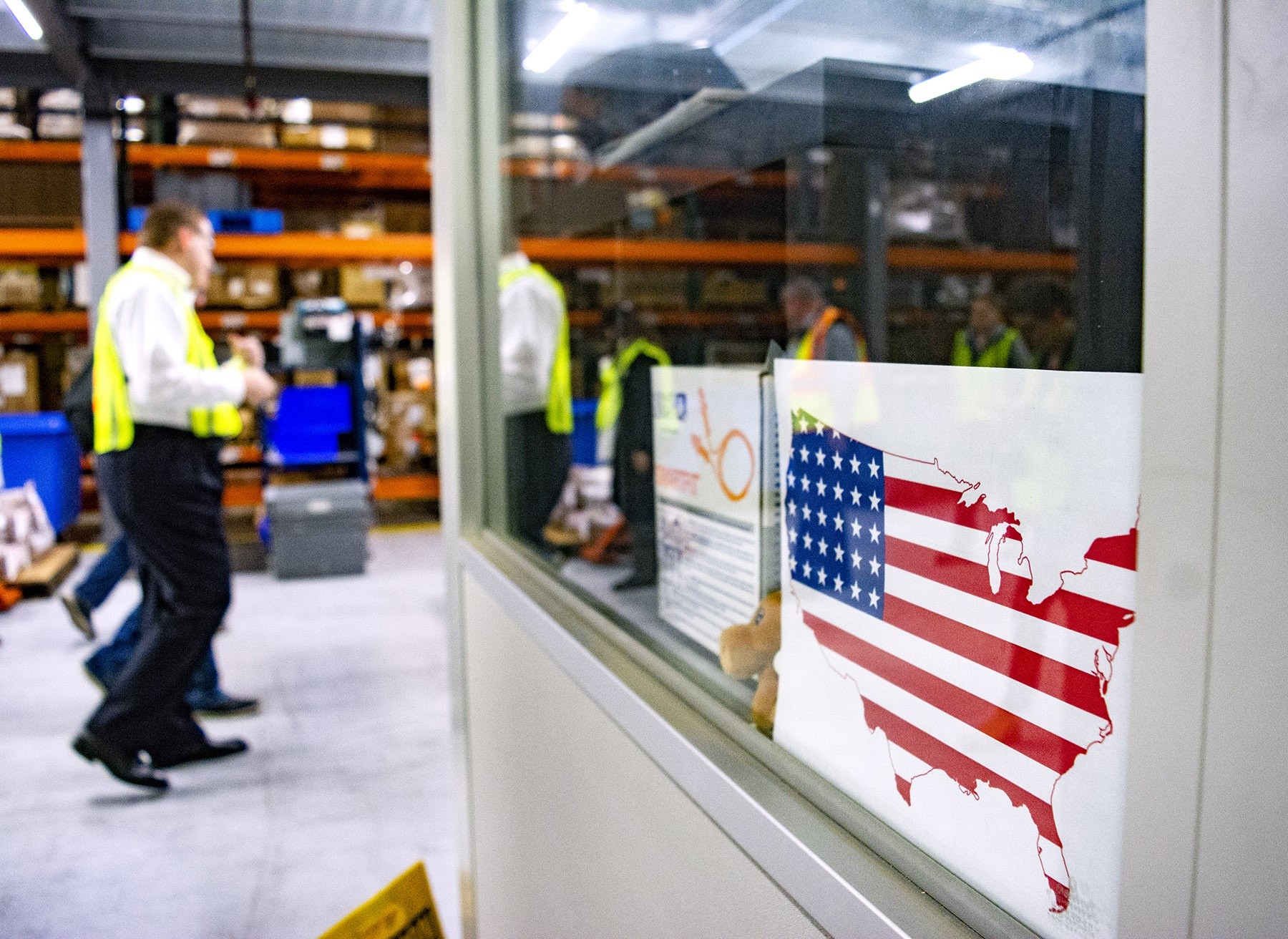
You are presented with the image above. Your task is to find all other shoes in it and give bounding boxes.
[61,590,94,641]
[612,572,654,591]
[85,657,112,699]
[185,692,259,716]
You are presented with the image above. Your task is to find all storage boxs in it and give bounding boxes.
[649,365,780,654]
[0,86,381,579]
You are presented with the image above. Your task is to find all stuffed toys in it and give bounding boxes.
[720,587,781,734]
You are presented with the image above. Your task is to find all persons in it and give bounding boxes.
[495,232,574,566]
[59,201,280,792]
[783,278,867,363]
[594,307,673,588]
[951,293,1078,369]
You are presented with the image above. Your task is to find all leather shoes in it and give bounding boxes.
[153,730,245,767]
[72,729,167,788]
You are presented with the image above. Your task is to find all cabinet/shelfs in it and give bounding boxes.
[0,135,1076,504]
